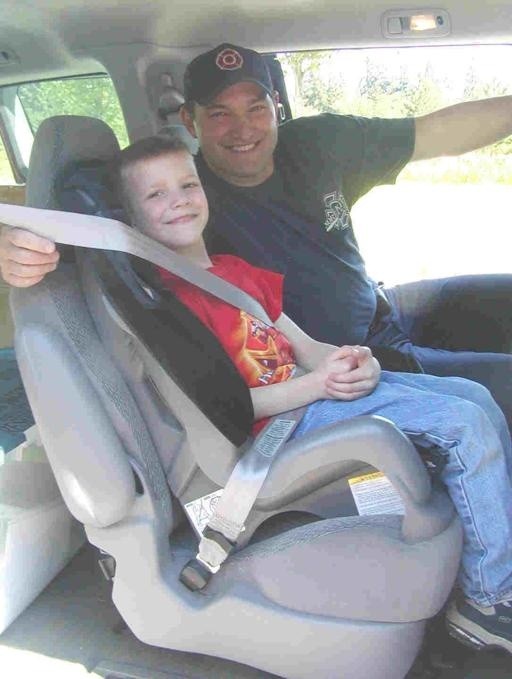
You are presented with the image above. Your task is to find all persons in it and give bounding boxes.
[108,137,512,658]
[1,41,511,430]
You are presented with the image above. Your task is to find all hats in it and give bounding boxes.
[183,41,275,108]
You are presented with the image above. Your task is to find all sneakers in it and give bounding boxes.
[444,592,512,656]
